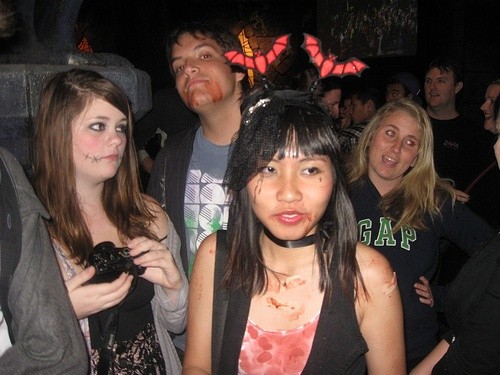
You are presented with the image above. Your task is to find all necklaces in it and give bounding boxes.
[263,227,316,248]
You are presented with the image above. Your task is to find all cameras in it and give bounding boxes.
[84,241,149,285]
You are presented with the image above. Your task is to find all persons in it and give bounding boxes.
[284,51,500,375]
[0,144,92,374]
[30,68,191,374]
[183,93,404,375]
[343,98,500,374]
[143,18,261,363]
